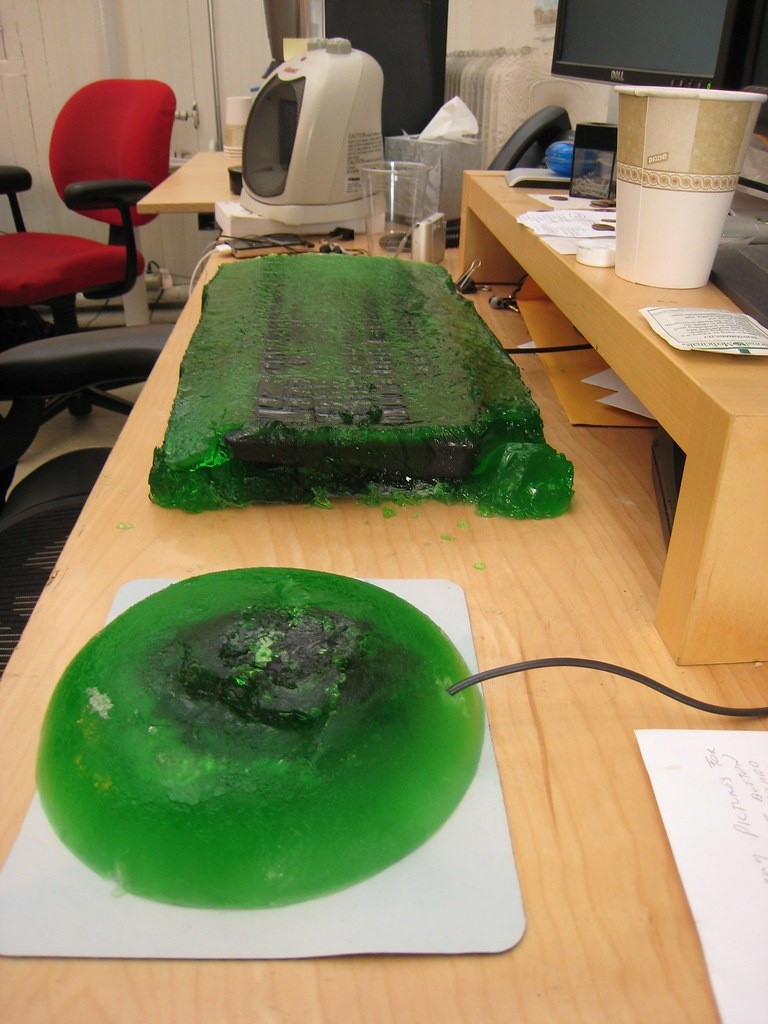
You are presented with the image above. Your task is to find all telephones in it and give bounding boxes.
[488,104,574,171]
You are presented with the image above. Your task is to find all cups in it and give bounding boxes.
[359,161,434,258]
[613,84,768,289]
[223,96,254,159]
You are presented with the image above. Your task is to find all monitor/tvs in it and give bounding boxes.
[319,0,450,140]
[506,0,757,188]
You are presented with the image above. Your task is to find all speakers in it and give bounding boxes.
[240,37,384,226]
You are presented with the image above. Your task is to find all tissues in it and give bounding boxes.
[384,96,482,229]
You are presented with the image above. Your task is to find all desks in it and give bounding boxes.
[136,149,242,230]
[0,171,768,1024]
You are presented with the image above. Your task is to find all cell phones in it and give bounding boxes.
[223,233,315,258]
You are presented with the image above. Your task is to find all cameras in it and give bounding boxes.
[412,213,446,264]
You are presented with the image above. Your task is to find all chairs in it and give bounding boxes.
[487,104,572,172]
[0,323,177,683]
[0,78,177,354]
